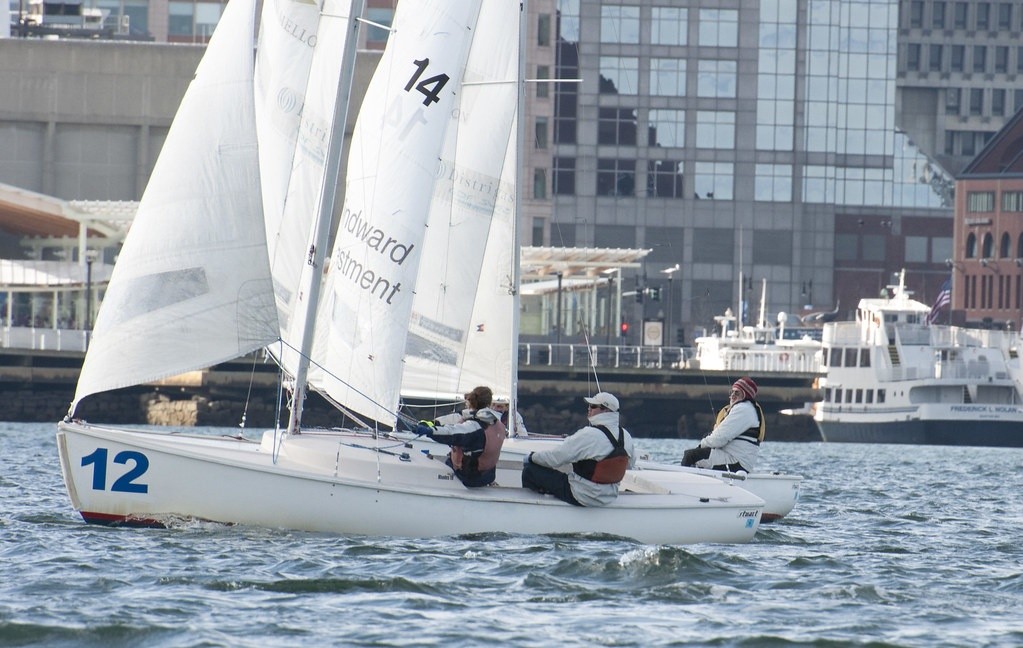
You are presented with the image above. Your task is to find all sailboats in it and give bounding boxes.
[56,0,805,547]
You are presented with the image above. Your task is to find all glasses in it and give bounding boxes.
[589,403,603,410]
[729,390,741,396]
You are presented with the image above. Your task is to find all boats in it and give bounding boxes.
[812,266,1023,450]
[693,223,828,372]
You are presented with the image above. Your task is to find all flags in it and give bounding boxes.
[926,273,951,326]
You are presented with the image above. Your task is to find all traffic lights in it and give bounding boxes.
[653,287,663,302]
[622,323,628,337]
[635,287,645,304]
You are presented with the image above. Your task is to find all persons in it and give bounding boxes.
[491,402,528,437]
[681,376,765,474]
[522,392,632,508]
[415,386,505,487]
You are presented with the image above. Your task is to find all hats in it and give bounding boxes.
[584,391,620,412]
[732,377,757,402]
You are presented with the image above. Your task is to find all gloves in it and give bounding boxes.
[523,452,533,463]
[413,425,433,437]
